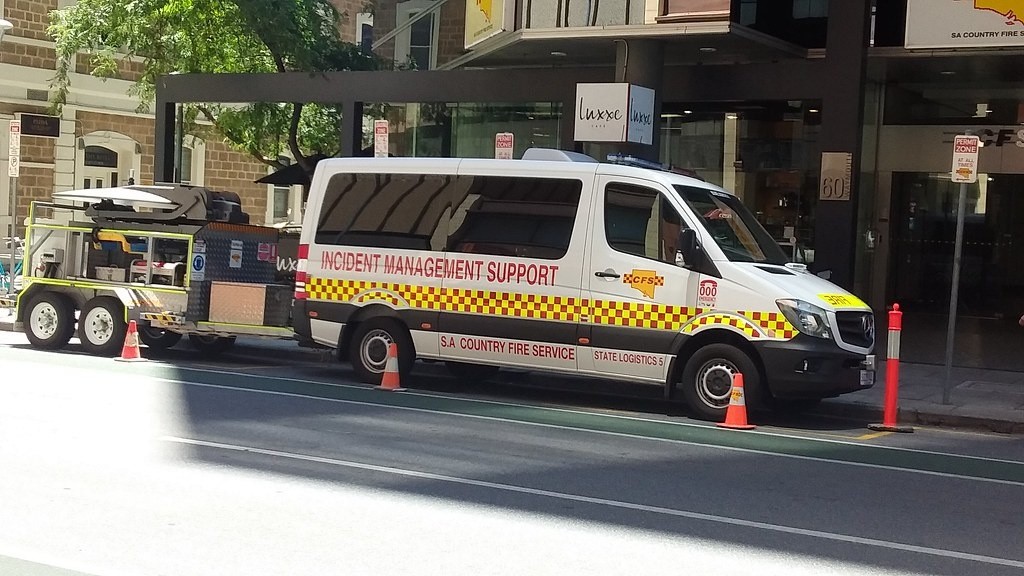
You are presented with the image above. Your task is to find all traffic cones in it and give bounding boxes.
[717,373,756,430]
[375,341,407,393]
[114,320,148,362]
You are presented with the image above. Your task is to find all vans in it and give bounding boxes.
[292,146,877,424]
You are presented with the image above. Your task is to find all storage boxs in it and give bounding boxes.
[209,280,293,328]
